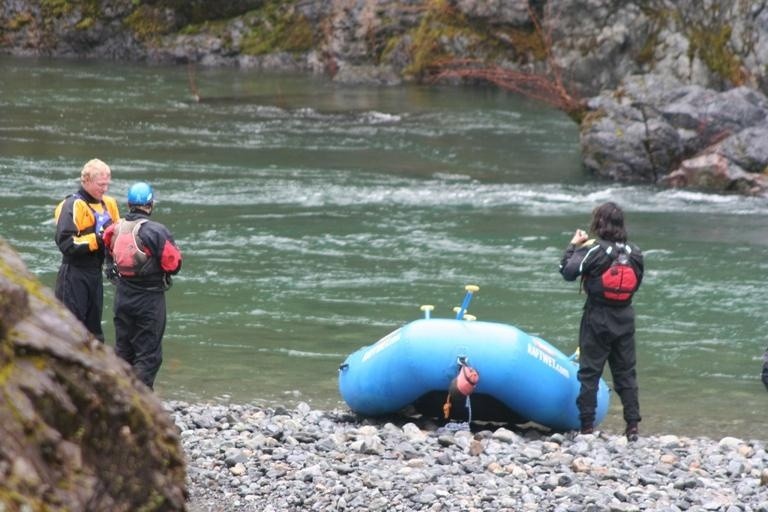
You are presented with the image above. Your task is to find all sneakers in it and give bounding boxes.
[627,425,638,441]
[582,420,593,433]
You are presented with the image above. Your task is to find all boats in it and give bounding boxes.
[337,314,610,432]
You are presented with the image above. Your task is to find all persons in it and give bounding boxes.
[53,158,120,344]
[103,182,183,387]
[559,201,643,442]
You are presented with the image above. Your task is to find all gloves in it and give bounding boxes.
[97,218,113,245]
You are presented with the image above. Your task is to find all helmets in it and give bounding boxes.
[127,183,153,206]
